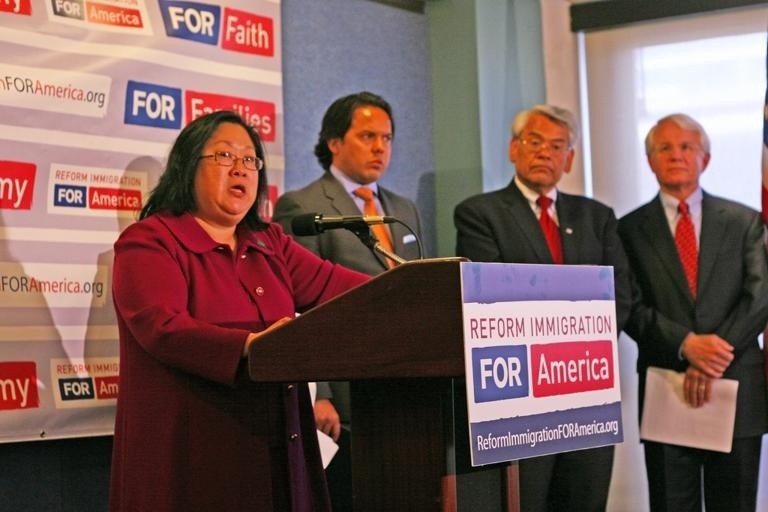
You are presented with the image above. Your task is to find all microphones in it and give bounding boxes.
[291,212,398,236]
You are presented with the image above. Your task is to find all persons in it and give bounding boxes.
[271,91,428,511]
[617,113,767,511]
[108,111,374,511]
[453,104,633,512]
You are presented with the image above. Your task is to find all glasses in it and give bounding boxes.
[199,151,265,172]
[518,136,570,154]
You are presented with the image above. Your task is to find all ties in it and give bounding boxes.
[353,186,397,269]
[675,201,700,302]
[535,194,566,265]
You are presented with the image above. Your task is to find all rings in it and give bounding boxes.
[698,384,706,390]
